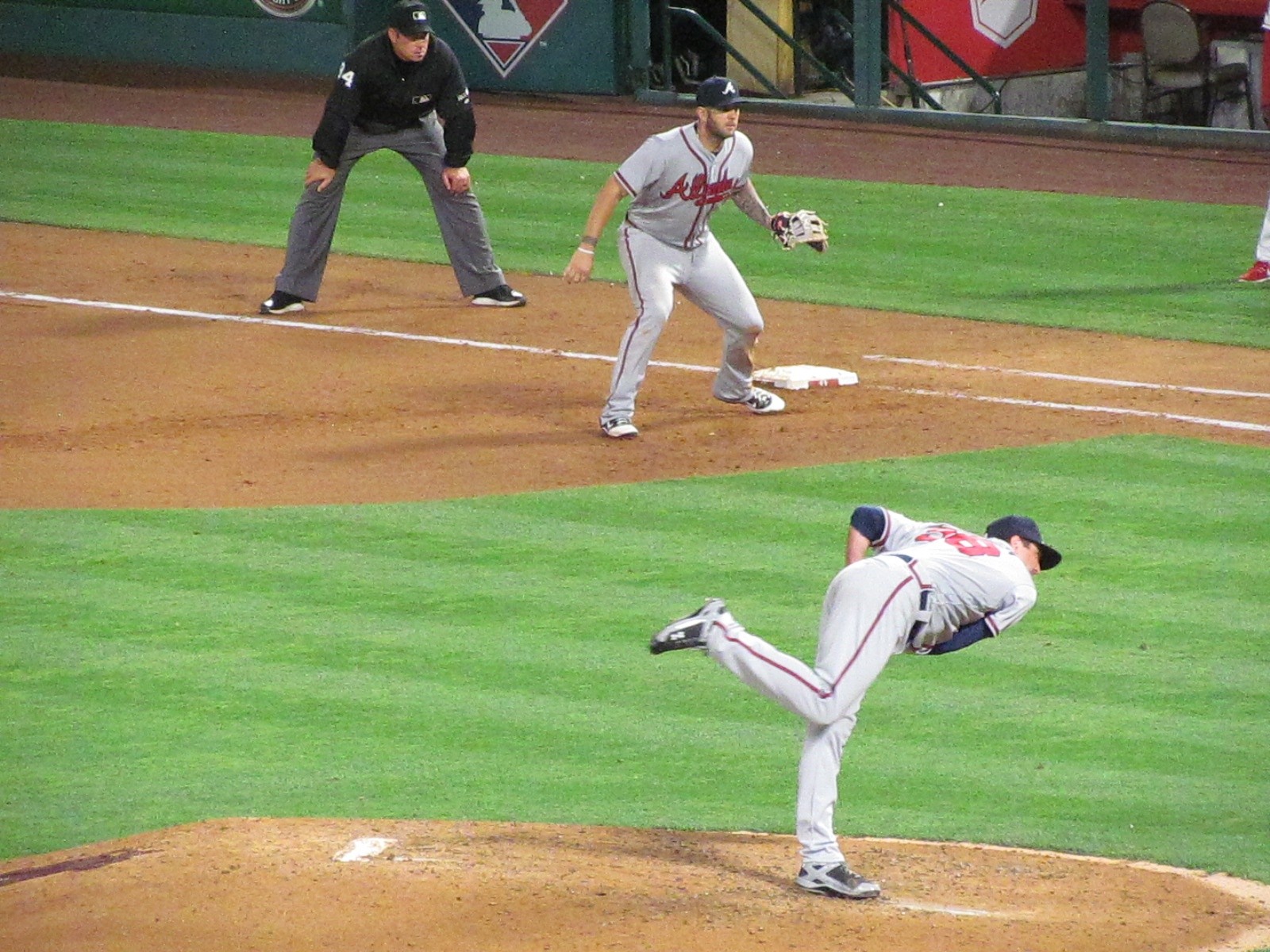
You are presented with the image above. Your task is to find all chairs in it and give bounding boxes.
[1138,0,1255,131]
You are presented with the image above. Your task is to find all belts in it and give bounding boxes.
[890,553,928,642]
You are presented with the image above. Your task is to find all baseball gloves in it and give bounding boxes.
[771,204,829,253]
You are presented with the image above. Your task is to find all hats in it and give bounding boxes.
[985,515,1063,571]
[696,76,747,107]
[389,0,436,35]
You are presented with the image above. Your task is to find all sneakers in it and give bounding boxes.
[652,597,728,655]
[714,386,785,413]
[600,416,638,439]
[1239,260,1270,283]
[261,290,305,314]
[796,861,881,899]
[472,285,526,308]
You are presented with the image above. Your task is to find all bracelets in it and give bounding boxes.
[577,247,595,254]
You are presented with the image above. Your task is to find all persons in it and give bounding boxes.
[650,503,1063,900]
[1238,5,1270,283]
[564,74,829,438]
[261,0,526,313]
